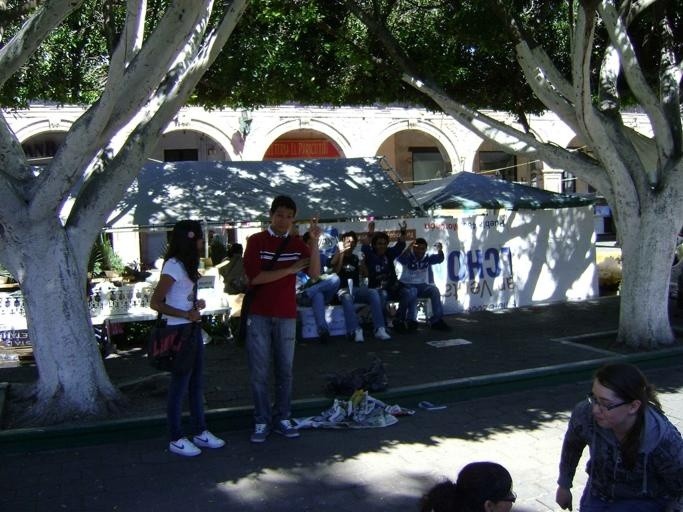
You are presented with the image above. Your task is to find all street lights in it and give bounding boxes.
[238,235,291,348]
[144,272,199,373]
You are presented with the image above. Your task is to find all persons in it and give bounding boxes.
[396,238,453,334]
[150,220,226,456]
[242,194,322,443]
[297,225,340,345]
[416,461,517,512]
[360,221,409,334]
[554,363,683,512]
[331,230,392,343]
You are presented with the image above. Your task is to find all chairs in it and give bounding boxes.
[272,416,301,438]
[373,327,392,341]
[431,320,453,333]
[192,431,226,449]
[170,437,201,456]
[251,423,272,443]
[355,328,365,343]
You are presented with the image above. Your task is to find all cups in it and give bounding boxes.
[585,393,630,410]
[494,490,517,504]
